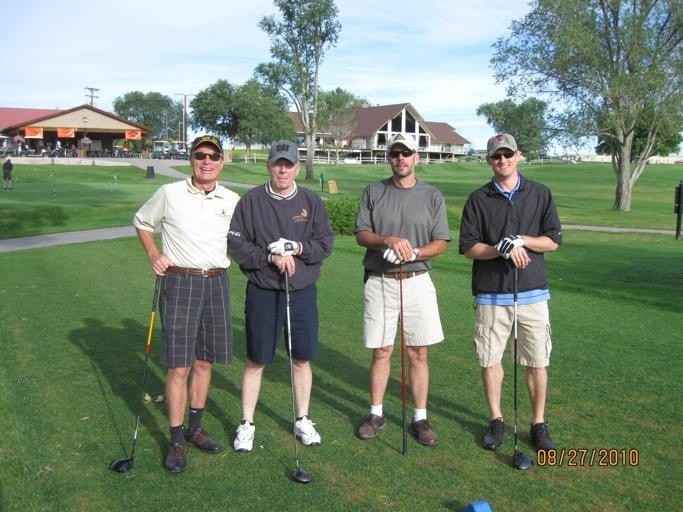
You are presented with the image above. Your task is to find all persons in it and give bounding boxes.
[457,133,561,456]
[3,159,13,189]
[353,132,452,445]
[71,143,78,158]
[0,136,63,157]
[132,135,242,473]
[122,140,130,151]
[227,139,334,452]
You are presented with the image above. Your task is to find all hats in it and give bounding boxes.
[388,132,418,156]
[190,136,223,153]
[266,140,300,165]
[487,133,517,156]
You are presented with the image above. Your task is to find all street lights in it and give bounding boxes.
[173,92,198,151]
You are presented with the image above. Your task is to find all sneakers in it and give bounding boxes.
[293,415,322,446]
[233,420,255,452]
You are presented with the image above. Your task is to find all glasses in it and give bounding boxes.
[389,148,413,158]
[190,153,223,161]
[490,150,517,160]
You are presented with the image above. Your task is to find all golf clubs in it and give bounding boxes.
[513,260,536,471]
[380,247,408,456]
[285,263,313,483]
[109,275,161,472]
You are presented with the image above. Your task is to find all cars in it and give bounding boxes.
[153,140,189,159]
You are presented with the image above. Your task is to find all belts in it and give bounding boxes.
[164,266,227,278]
[365,269,427,280]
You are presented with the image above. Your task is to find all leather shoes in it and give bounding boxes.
[481,419,504,450]
[163,440,188,474]
[408,416,439,445]
[356,412,384,439]
[528,421,556,455]
[184,427,222,455]
